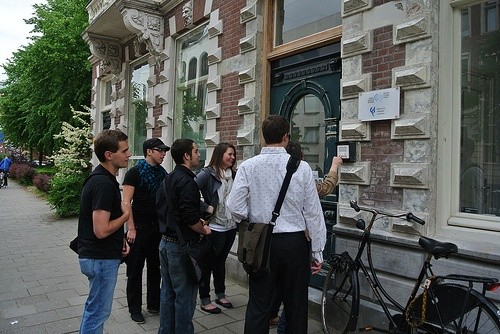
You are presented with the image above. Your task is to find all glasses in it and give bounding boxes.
[153,148,166,153]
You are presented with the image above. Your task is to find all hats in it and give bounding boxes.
[143,138,170,153]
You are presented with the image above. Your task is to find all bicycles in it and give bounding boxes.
[321,200,500,334]
[0,170,6,188]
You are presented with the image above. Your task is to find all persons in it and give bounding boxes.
[194,142,236,314]
[77,129,130,334]
[227,115,326,334]
[123,138,169,323]
[0,156,11,188]
[269,141,342,326]
[157,138,212,334]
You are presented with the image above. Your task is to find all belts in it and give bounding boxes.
[161,234,181,243]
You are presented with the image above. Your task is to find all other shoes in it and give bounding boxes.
[268,316,280,328]
[215,298,235,309]
[148,308,161,314]
[201,305,221,313]
[130,313,145,324]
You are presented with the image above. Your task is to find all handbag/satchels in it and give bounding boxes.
[4,171,10,178]
[185,239,216,284]
[69,237,80,255]
[237,222,273,275]
